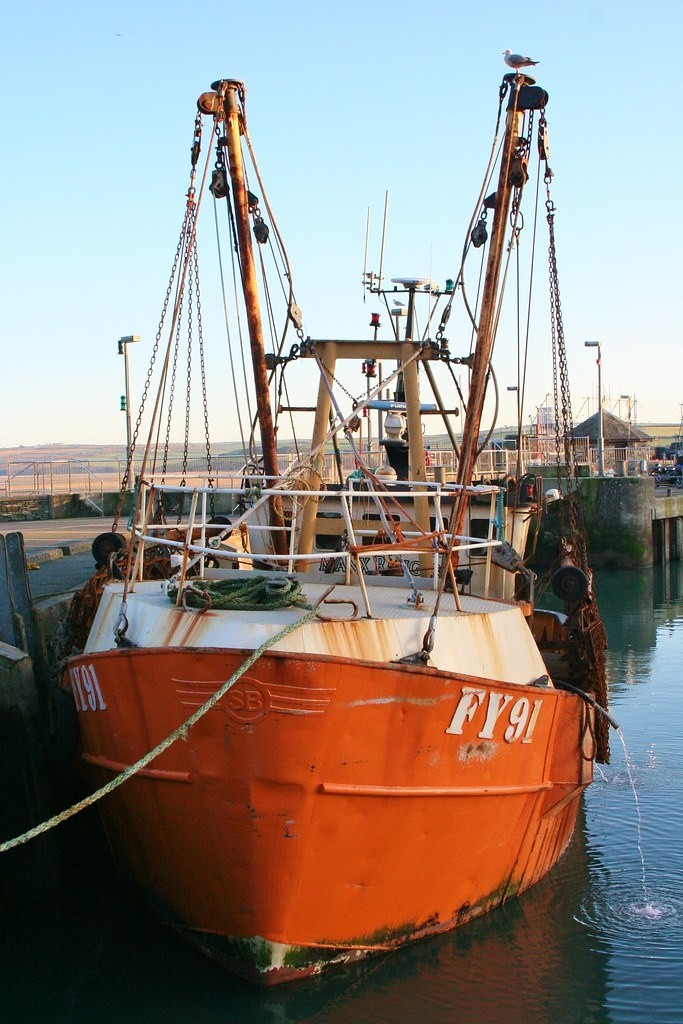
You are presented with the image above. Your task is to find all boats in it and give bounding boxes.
[61,70,609,1001]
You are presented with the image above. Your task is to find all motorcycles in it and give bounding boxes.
[651,462,683,490]
